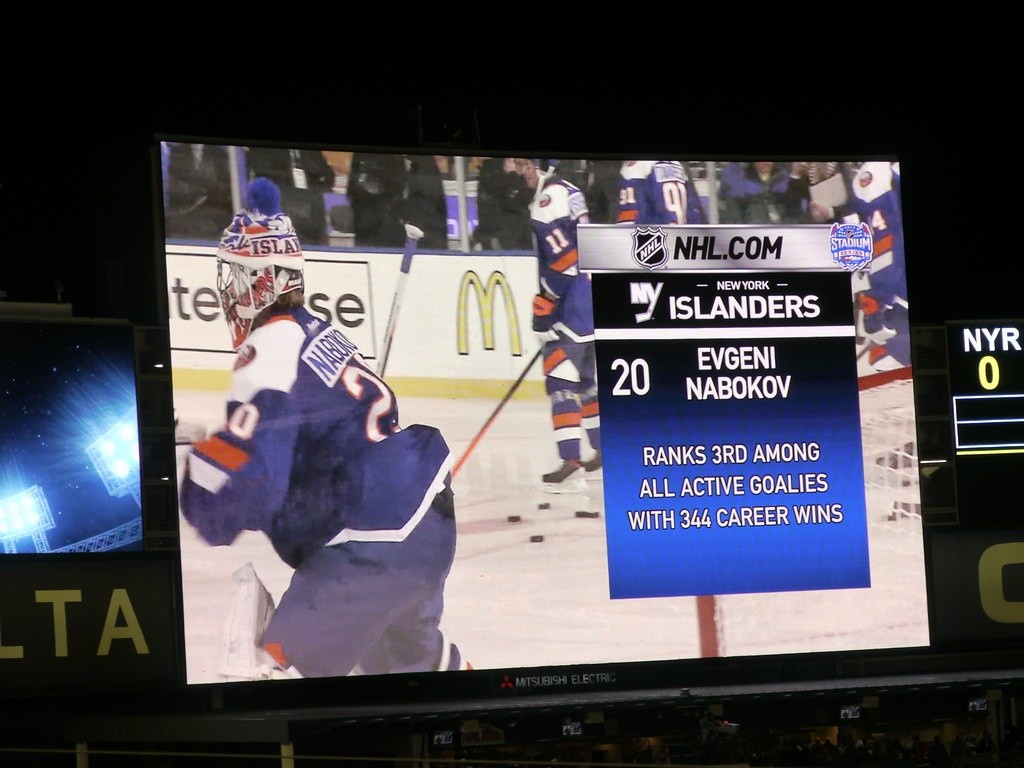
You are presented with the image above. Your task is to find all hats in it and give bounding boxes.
[218,175,308,354]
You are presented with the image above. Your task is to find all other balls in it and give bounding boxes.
[529,534,544,541]
[508,516,521,522]
[538,501,550,509]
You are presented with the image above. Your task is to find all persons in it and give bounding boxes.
[164,140,911,690]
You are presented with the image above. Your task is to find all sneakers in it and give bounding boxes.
[584,449,602,481]
[539,457,590,493]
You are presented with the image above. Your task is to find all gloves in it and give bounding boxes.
[863,302,899,347]
[535,328,561,349]
